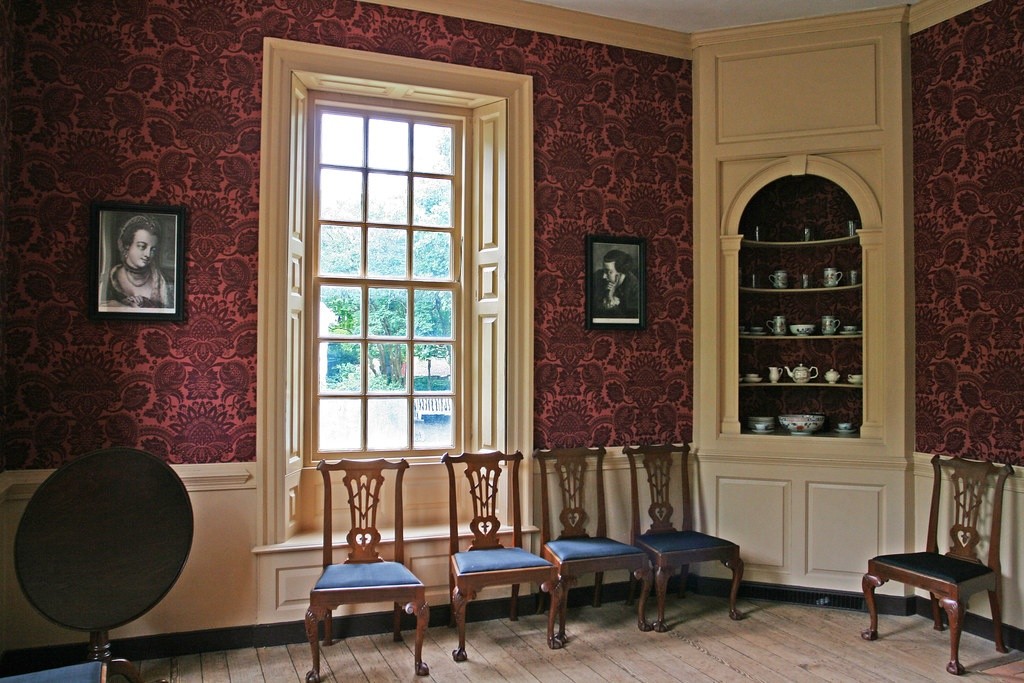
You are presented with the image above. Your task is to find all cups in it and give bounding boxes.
[755,221,856,242]
[768,268,789,289]
[750,327,763,332]
[839,423,850,430]
[817,267,843,287]
[845,326,857,331]
[739,326,745,332]
[824,368,840,384]
[768,367,783,383]
[767,315,786,336]
[745,373,758,378]
[822,315,840,334]
[847,375,863,379]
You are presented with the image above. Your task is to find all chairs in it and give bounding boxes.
[622,441,746,633]
[440,449,562,662]
[861,454,1014,676]
[305,458,429,683]
[532,446,653,643]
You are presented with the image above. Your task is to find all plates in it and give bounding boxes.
[743,378,763,382]
[751,332,765,335]
[840,332,857,334]
[739,332,750,334]
[848,379,862,384]
[834,429,857,432]
[752,429,774,433]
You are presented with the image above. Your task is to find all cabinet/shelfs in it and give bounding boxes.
[739,235,860,389]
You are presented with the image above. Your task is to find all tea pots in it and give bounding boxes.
[784,364,818,383]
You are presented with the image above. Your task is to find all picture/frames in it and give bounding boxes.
[92,203,187,321]
[584,233,648,330]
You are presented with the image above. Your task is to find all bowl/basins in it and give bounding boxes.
[747,416,774,429]
[789,323,816,336]
[739,378,743,382]
[778,415,824,435]
